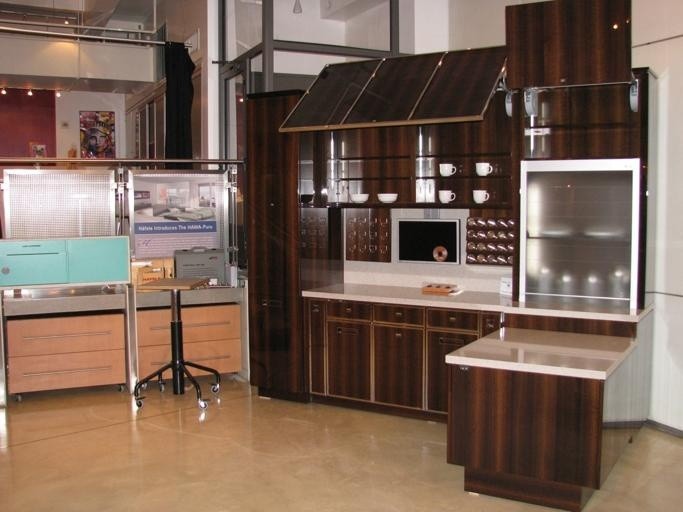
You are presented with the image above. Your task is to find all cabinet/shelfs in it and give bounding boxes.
[2,288,127,401]
[131,283,241,389]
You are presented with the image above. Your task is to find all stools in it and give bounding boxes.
[136,277,220,409]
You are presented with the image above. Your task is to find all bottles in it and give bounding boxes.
[66,147,93,158]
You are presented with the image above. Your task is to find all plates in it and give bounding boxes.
[540,226,570,236]
[584,227,619,240]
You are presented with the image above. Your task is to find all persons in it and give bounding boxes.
[87,126,113,159]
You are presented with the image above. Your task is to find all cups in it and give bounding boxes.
[437,163,456,177]
[471,189,489,203]
[466,217,514,264]
[533,265,629,308]
[436,189,456,205]
[474,162,493,175]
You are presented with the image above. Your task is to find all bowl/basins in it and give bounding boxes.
[348,193,370,204]
[376,193,399,204]
